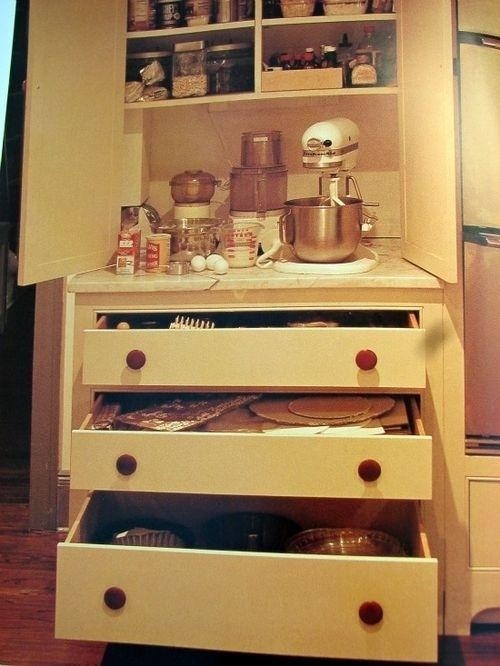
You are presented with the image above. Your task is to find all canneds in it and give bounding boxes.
[145,233,171,273]
[158,0,181,27]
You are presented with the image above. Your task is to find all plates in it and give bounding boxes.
[99,510,406,557]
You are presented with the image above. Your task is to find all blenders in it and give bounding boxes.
[270,116,380,275]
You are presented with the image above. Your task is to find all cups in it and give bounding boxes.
[222,222,265,268]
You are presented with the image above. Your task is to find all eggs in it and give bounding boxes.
[191,254,228,275]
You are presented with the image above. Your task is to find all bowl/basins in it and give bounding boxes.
[154,218,225,262]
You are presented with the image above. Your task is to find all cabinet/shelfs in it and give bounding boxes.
[124,0,399,111]
[53,291,445,664]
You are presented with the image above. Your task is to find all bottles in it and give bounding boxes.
[126,37,255,99]
[168,167,222,202]
[278,32,385,87]
